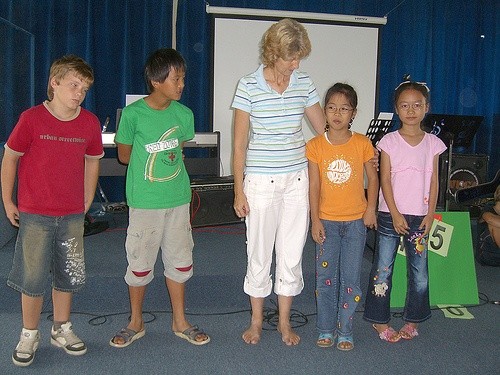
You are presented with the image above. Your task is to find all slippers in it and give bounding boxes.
[317,333,336,347]
[174,323,210,346]
[337,334,354,351]
[372,322,400,342]
[108,326,147,348]
[399,322,418,340]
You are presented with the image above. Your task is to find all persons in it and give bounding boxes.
[109,48,212,349]
[1,53,104,367]
[364,81,447,343]
[477,199,500,267]
[304,84,379,350]
[231,19,380,346]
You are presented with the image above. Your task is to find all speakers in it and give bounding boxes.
[438,152,490,208]
[188,178,245,227]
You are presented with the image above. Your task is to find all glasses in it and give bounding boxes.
[397,103,426,110]
[325,104,354,114]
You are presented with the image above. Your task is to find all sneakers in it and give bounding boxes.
[10,328,41,367]
[50,320,87,356]
